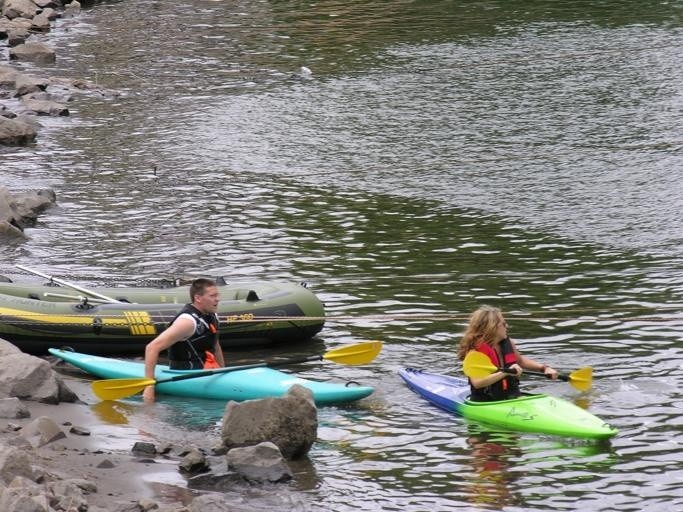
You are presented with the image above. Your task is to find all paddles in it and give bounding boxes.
[463,351,592,392]
[90,341,382,401]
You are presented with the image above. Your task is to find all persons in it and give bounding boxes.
[455,303,561,402]
[141,277,226,404]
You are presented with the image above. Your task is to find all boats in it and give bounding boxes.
[0,281,328,350]
[399,365,621,442]
[46,347,376,407]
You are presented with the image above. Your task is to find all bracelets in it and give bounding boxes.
[540,364,550,374]
